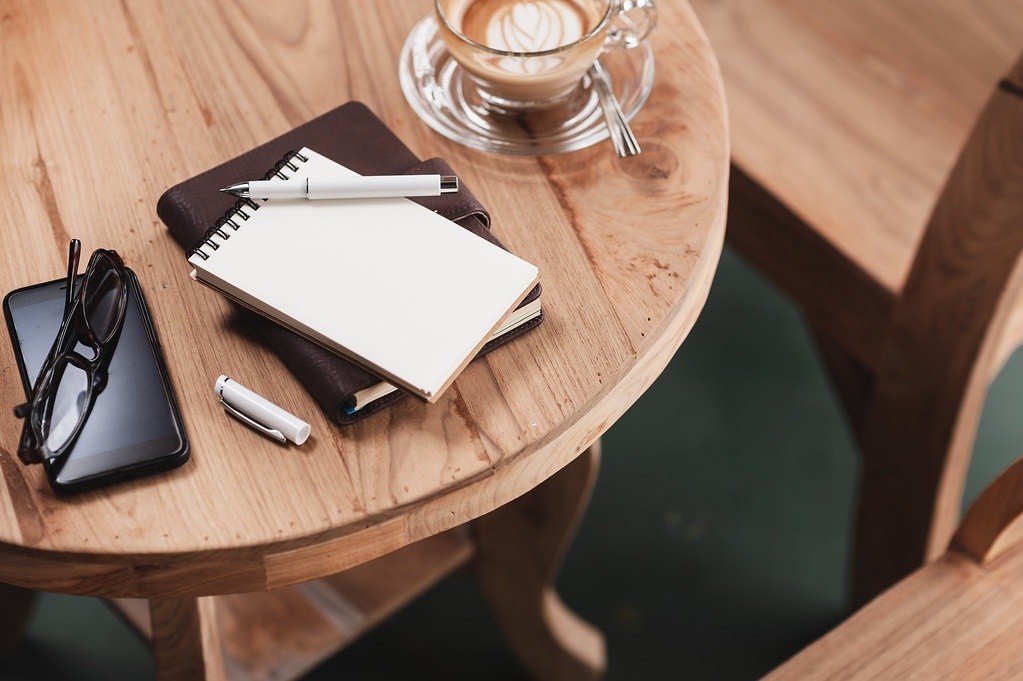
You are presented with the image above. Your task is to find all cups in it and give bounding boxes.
[431,0,658,116]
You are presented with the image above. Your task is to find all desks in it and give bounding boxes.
[0,1,733,680]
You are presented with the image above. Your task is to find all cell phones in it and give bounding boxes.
[1,266,191,494]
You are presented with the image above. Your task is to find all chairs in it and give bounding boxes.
[689,0,1022,681]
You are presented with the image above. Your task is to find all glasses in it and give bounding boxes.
[14,238,130,465]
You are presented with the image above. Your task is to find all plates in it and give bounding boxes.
[398,7,654,156]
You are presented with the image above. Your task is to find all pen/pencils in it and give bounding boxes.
[219,174,459,201]
